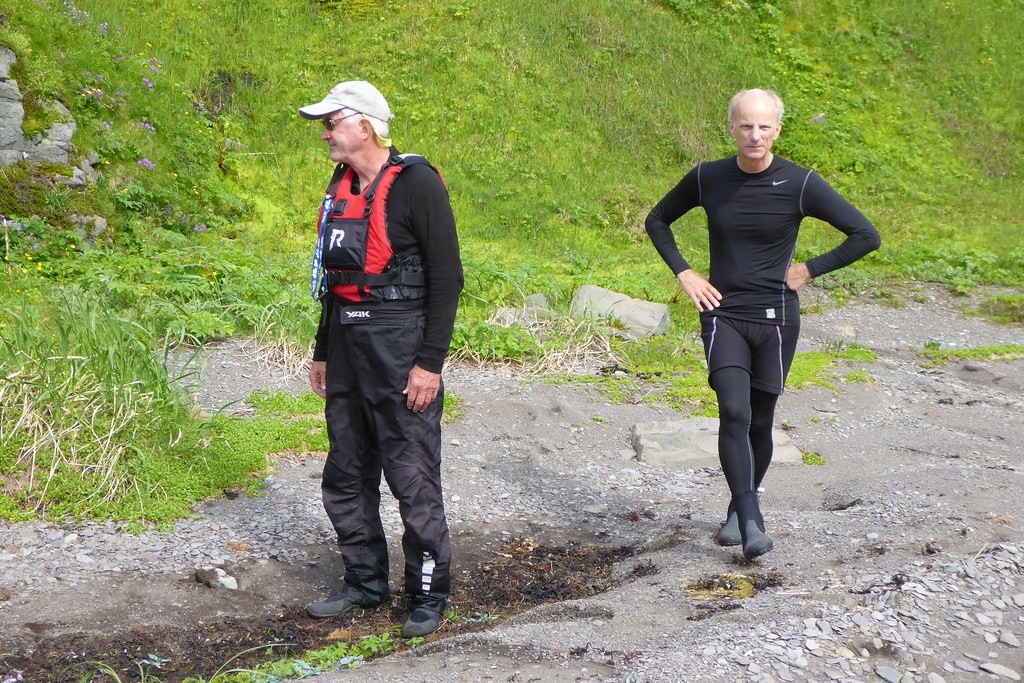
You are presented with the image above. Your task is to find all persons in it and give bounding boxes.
[308,80,464,638]
[642,86,879,558]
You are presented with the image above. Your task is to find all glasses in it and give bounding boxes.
[321,112,362,130]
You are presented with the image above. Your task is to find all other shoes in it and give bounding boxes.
[401,607,442,638]
[306,593,361,619]
[717,499,742,546]
[739,514,774,560]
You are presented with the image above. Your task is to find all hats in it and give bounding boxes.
[299,80,390,122]
